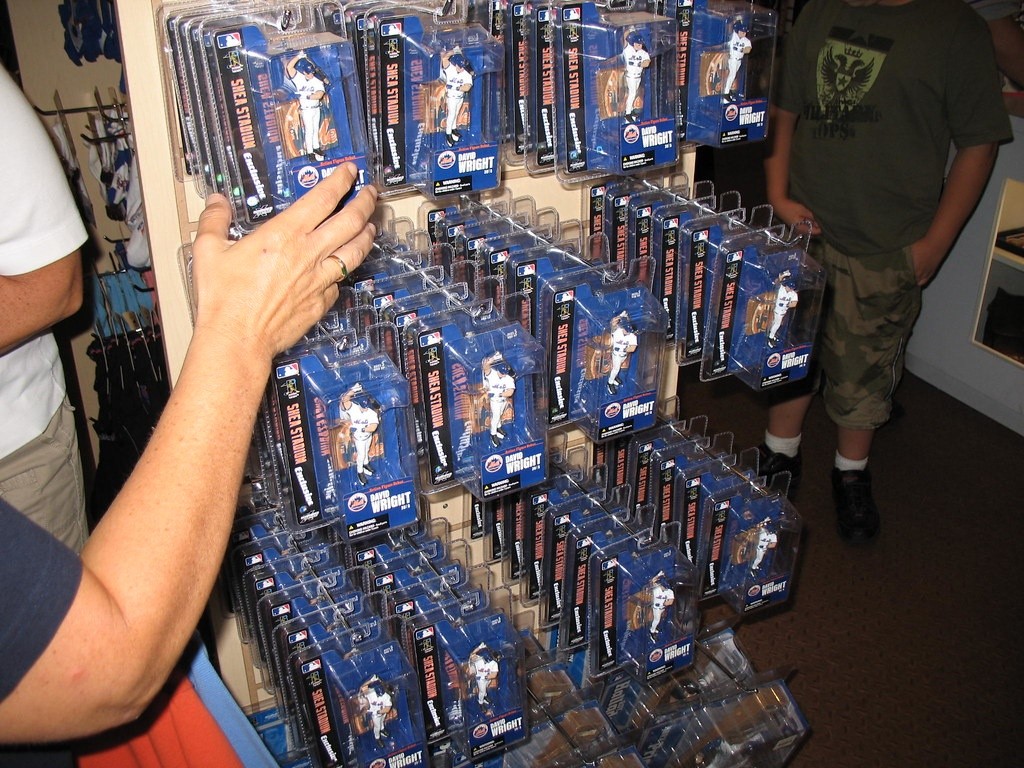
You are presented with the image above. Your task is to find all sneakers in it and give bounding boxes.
[752,441,802,490]
[833,462,884,546]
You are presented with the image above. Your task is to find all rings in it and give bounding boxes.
[327,255,347,283]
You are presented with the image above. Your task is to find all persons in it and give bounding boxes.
[751,0,1024,546]
[649,571,675,642]
[607,310,638,394]
[751,517,777,574]
[0,58,87,552]
[443,50,473,143]
[342,383,379,483]
[360,674,392,748]
[767,270,798,347]
[287,50,326,163]
[482,351,516,446]
[623,25,651,123]
[0,163,378,768]
[723,16,753,101]
[469,641,499,709]
